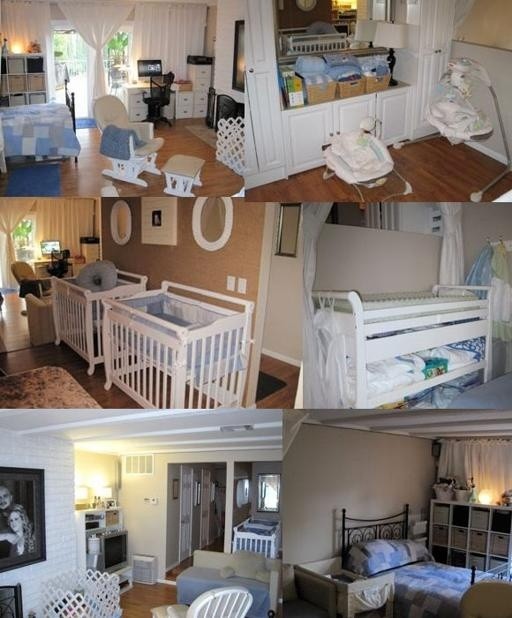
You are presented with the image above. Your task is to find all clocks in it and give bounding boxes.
[295,0,317,12]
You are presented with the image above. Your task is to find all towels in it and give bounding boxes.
[99,123,148,161]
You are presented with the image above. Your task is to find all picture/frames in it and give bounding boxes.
[195,482,200,506]
[0,466,46,573]
[275,202,302,257]
[232,20,245,92]
[141,197,177,245]
[173,479,179,499]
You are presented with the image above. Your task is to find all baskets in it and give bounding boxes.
[433,525,448,545]
[366,74,391,94]
[470,555,485,571]
[470,531,488,553]
[433,506,449,523]
[337,76,366,99]
[490,534,509,556]
[471,511,489,530]
[307,82,337,105]
[489,559,506,570]
[452,528,467,549]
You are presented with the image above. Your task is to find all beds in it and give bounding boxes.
[233,516,282,558]
[283,557,395,618]
[0,80,81,163]
[342,504,512,618]
[101,280,255,409]
[51,267,148,374]
[310,284,493,409]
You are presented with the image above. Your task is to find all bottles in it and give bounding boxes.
[93,496,97,509]
[97,497,101,509]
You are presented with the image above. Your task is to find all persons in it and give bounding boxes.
[7,504,36,558]
[0,486,21,559]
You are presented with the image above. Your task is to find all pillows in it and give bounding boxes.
[222,551,270,583]
[345,539,434,576]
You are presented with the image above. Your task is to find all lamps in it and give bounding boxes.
[354,18,383,48]
[373,22,408,86]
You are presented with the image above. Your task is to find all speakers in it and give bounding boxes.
[41,240,62,257]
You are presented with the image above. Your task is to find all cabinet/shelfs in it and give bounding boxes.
[1,53,47,107]
[430,498,512,576]
[164,63,211,120]
[246,0,286,191]
[281,79,413,177]
[394,0,455,140]
[75,505,133,595]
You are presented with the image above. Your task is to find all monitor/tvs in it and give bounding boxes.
[97,530,129,573]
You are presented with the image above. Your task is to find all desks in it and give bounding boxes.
[124,83,175,121]
[34,257,85,277]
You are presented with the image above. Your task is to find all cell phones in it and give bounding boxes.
[137,60,162,76]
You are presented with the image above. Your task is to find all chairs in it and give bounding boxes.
[48,250,69,279]
[93,94,165,187]
[11,261,52,315]
[151,586,253,618]
[141,73,175,128]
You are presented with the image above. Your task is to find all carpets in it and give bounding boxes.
[185,125,218,150]
[7,163,61,197]
[256,370,287,401]
[0,366,102,409]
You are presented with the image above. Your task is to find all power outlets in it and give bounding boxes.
[227,276,235,290]
[238,278,246,293]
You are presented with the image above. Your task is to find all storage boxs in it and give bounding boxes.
[81,244,98,264]
[284,74,391,108]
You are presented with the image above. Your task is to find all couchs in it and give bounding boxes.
[175,550,282,618]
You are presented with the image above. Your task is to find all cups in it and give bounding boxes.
[88,538,100,554]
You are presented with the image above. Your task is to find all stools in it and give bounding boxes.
[26,294,56,346]
[161,154,205,197]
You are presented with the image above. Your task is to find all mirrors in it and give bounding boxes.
[108,200,132,246]
[190,197,235,252]
[273,0,394,63]
[256,472,280,513]
